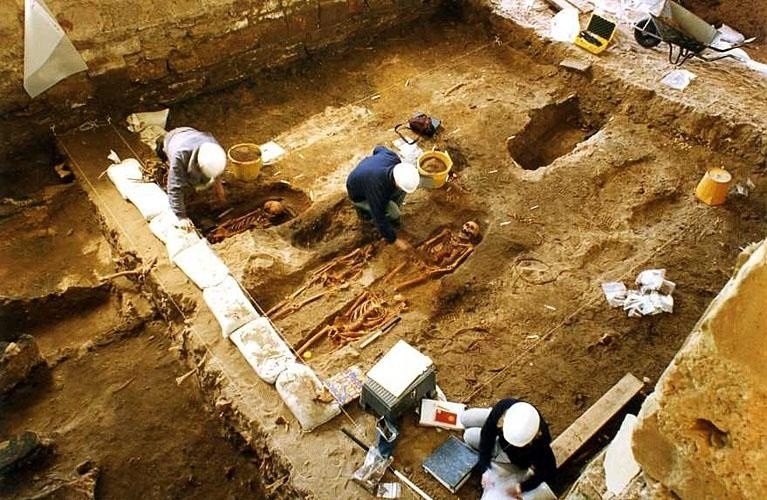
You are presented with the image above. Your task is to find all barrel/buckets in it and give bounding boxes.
[416,150,453,189]
[227,143,262,182]
[695,166,732,207]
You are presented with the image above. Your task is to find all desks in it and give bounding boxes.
[359,367,437,429]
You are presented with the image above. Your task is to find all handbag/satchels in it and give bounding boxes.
[409,114,435,136]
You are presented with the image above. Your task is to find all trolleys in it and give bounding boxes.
[632,0,761,70]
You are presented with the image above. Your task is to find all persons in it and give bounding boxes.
[461,398,557,500]
[153,123,228,220]
[344,144,424,253]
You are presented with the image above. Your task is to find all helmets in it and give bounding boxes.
[197,142,227,178]
[503,402,540,448]
[393,163,420,194]
